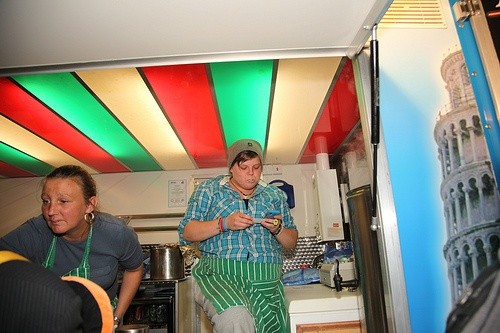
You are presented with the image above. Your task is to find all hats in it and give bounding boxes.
[227,139,264,174]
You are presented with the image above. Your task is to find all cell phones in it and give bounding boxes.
[265,210,280,218]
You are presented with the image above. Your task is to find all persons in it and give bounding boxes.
[0,165,144,333]
[177,139,298,333]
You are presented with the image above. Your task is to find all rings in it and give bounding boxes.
[274,219,278,226]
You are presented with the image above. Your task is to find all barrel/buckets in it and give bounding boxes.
[151,248,184,279]
[115,324,149,333]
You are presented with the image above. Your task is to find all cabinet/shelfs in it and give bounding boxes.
[283,295,363,333]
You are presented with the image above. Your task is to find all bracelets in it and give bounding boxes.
[271,219,283,237]
[217,216,228,234]
[113,316,121,326]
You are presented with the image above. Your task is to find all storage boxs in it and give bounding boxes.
[319,261,355,287]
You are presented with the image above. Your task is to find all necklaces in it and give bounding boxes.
[229,178,255,199]
